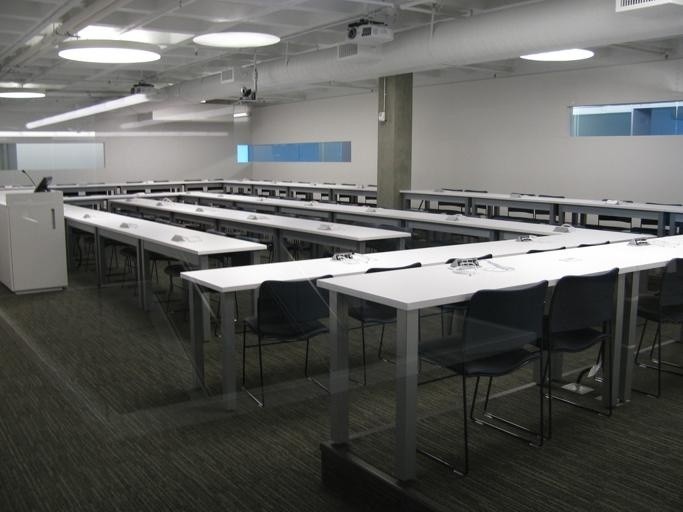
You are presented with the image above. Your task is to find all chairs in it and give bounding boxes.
[481,268,619,442]
[240,273,335,410]
[345,262,427,387]
[36,177,683,308]
[598,257,683,400]
[414,280,551,481]
[433,254,494,338]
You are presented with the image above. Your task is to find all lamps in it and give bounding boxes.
[0,23,282,130]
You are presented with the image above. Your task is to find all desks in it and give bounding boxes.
[180,235,659,412]
[315,253,630,493]
[140,227,268,343]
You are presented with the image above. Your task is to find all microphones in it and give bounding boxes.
[21,169,37,187]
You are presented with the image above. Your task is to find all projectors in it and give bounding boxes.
[131,87,156,95]
[346,25,394,43]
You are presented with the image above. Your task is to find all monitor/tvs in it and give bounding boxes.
[34,176,53,192]
